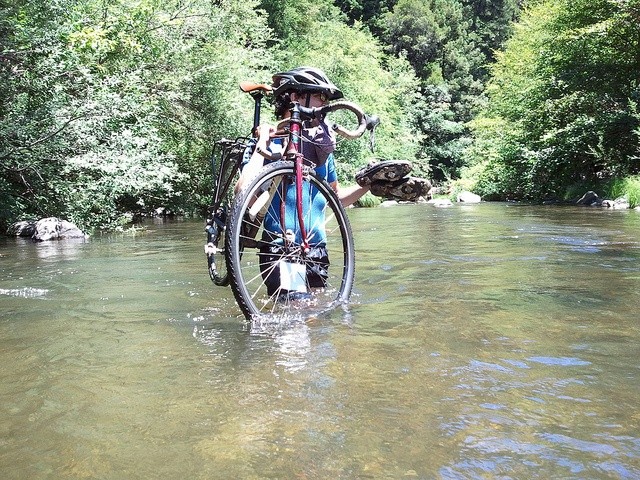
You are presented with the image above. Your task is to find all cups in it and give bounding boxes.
[312,90,330,105]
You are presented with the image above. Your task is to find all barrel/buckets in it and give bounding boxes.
[355,157,412,187]
[370,176,432,202]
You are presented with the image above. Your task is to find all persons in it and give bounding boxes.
[234,66,379,315]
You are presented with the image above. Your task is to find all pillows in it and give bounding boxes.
[271,66,344,101]
